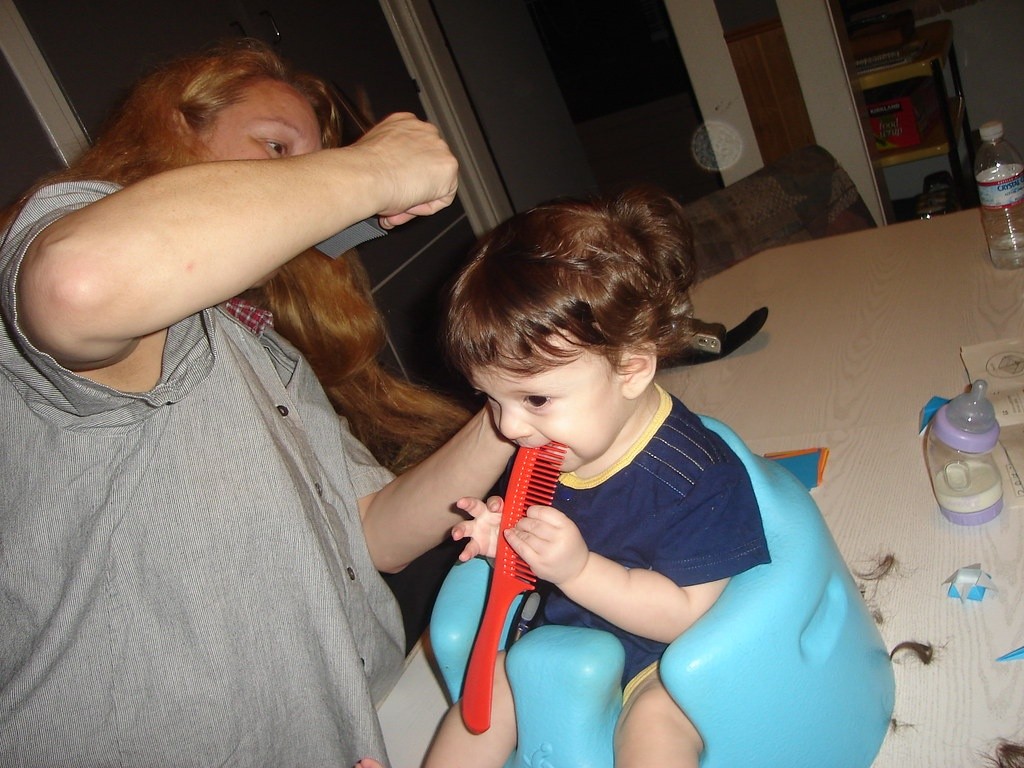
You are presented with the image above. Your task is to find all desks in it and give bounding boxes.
[376,211,1024,768]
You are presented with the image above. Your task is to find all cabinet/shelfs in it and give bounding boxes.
[863,19,978,210]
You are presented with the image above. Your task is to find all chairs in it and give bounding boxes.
[687,143,875,285]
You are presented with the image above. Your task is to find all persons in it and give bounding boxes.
[0,37,517,768]
[354,190,771,768]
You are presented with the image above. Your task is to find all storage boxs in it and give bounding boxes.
[862,75,942,154]
[850,8,917,56]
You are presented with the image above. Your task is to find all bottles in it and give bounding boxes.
[927,380,1004,525]
[974,121,1024,270]
